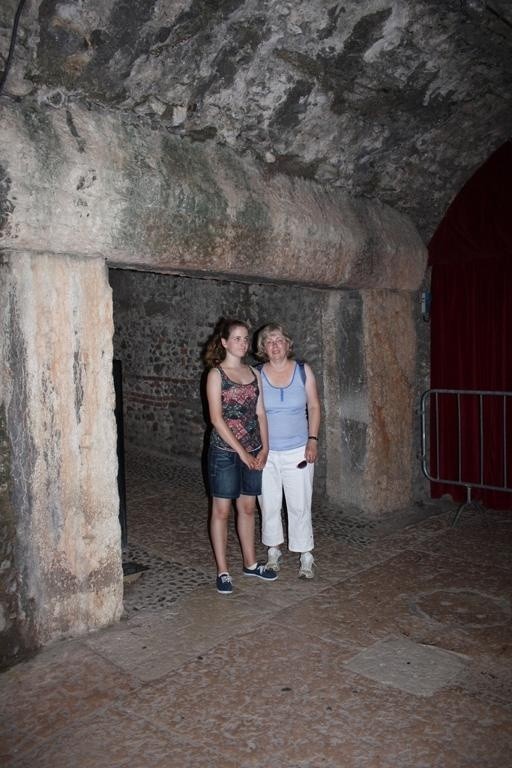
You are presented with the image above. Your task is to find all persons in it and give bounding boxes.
[201,316,321,594]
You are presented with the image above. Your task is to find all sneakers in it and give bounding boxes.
[243,546,283,581]
[297,552,313,579]
[215,574,233,594]
